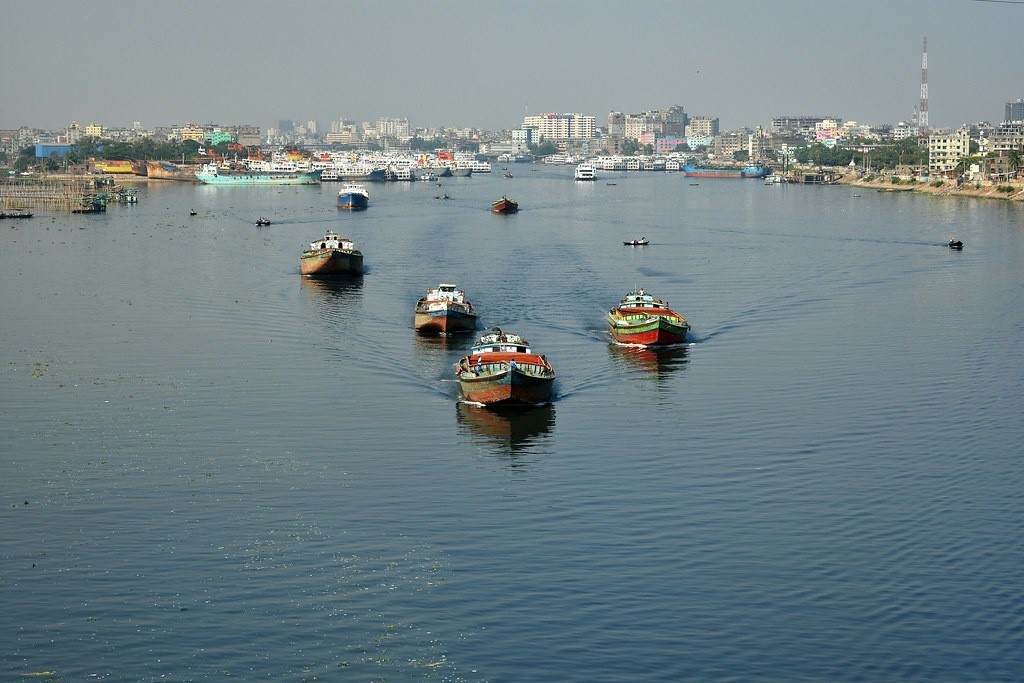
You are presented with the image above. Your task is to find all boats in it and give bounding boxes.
[300,229,364,276]
[623,237,650,246]
[491,196,519,211]
[456,394,556,453]
[414,284,477,336]
[6,213,34,218]
[606,284,691,345]
[682,160,773,178]
[455,327,557,406]
[948,238,963,249]
[338,180,369,211]
[72,197,107,213]
[144,158,247,180]
[129,159,211,176]
[195,147,492,185]
[764,175,787,182]
[875,188,914,192]
[575,162,598,180]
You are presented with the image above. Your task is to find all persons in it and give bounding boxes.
[666,302,669,309]
[640,287,645,295]
[477,354,484,373]
[510,358,517,366]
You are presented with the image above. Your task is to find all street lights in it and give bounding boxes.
[786,151,789,174]
[782,151,784,174]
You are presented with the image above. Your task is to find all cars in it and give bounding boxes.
[885,170,895,175]
